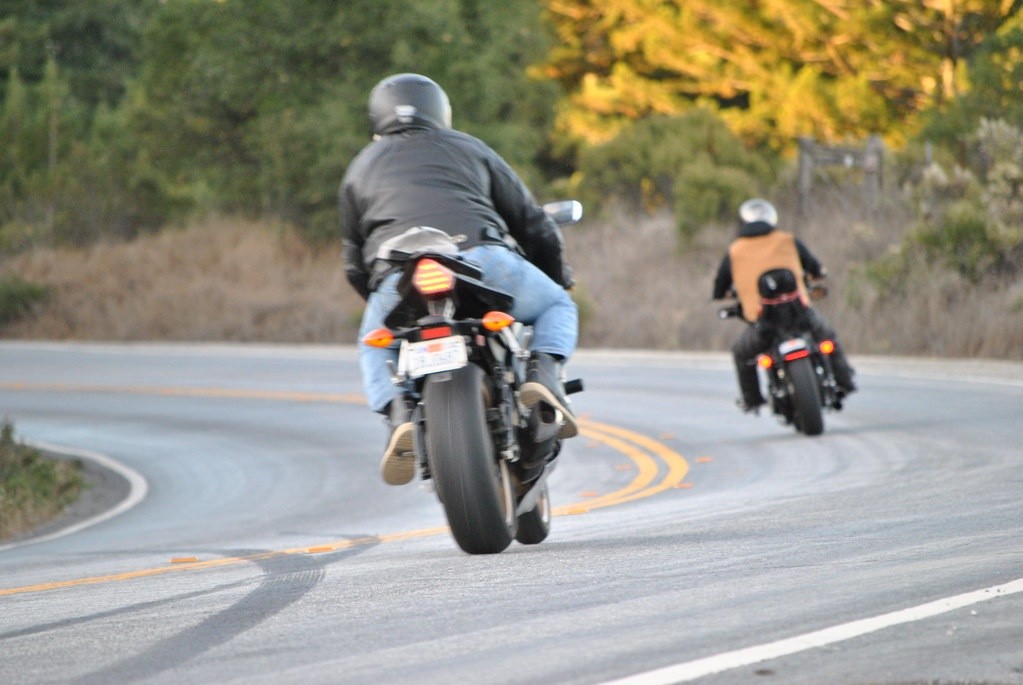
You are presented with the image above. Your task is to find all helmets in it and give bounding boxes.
[366,72,451,138]
[738,197,779,232]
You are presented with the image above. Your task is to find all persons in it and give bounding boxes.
[337,73,579,487]
[713,197,855,409]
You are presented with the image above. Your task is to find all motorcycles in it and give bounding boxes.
[719,265,851,437]
[361,198,583,554]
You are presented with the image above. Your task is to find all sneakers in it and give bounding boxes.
[518,351,580,439]
[379,392,417,485]
